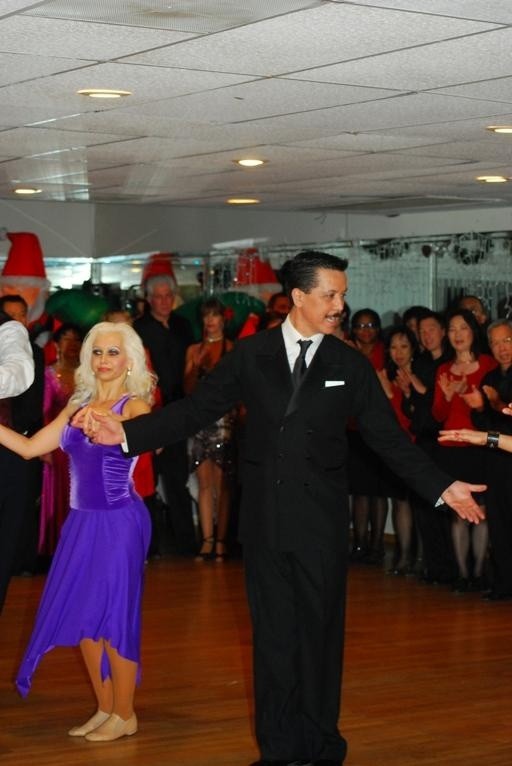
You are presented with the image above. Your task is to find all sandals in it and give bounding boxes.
[195,537,227,565]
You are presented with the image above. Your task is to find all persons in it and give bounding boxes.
[3,295,82,603]
[131,273,290,563]
[70,247,492,765]
[1,318,161,746]
[344,295,511,602]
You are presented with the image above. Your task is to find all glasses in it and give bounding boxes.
[489,336,512,348]
[356,322,377,329]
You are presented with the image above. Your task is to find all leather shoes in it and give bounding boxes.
[86,713,139,742]
[68,710,109,737]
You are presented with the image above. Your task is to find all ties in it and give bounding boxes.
[292,340,311,390]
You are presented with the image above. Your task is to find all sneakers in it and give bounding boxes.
[352,548,510,601]
